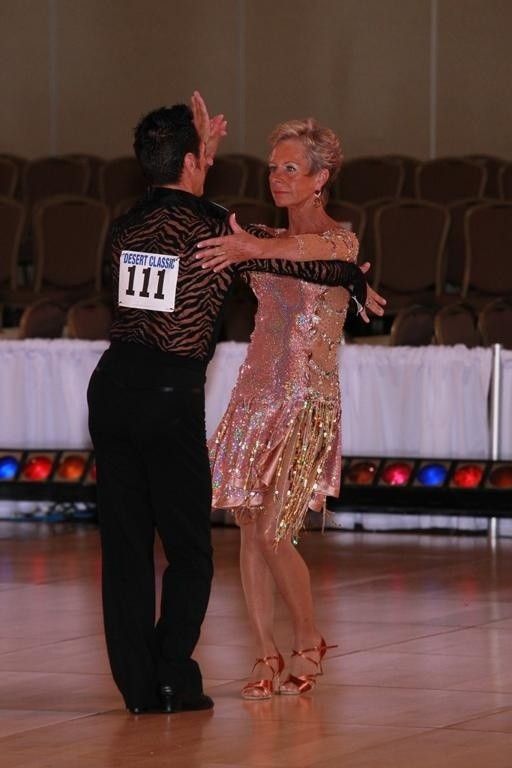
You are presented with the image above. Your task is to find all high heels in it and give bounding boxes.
[240,648,284,700]
[279,637,338,695]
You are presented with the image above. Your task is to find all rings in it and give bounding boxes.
[366,296,372,307]
[210,123,217,128]
[214,247,226,256]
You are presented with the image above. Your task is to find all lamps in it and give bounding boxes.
[323,456,512,520]
[0,444,101,519]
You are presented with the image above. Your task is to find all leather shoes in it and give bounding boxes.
[158,683,213,713]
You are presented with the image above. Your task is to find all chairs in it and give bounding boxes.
[0,151,512,343]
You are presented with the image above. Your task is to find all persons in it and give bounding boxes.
[84,89,390,716]
[195,112,361,700]
